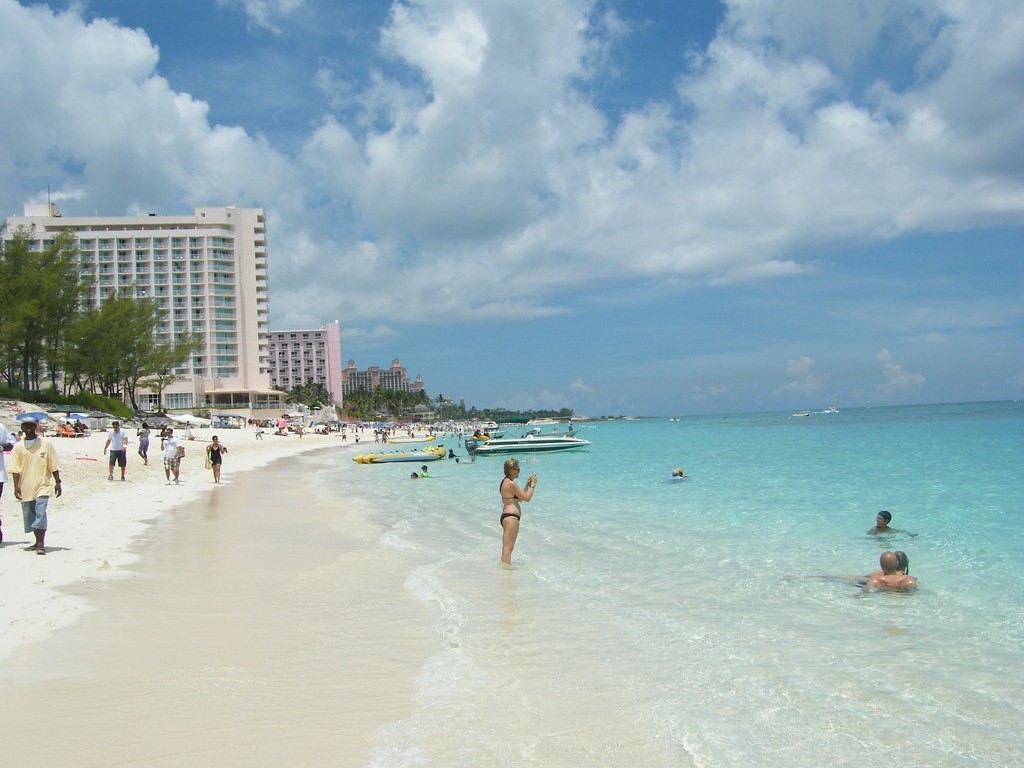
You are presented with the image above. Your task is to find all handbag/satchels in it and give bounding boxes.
[204,453,214,470]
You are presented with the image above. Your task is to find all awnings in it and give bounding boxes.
[283,412,305,424]
[16,410,48,422]
[213,413,247,430]
[87,412,110,432]
[60,412,87,425]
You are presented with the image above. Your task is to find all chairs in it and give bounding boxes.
[186,427,208,442]
[58,425,84,439]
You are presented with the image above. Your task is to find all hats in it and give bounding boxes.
[21,416,38,432]
[449,449,454,452]
[421,465,427,469]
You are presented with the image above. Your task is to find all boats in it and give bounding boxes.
[460,430,506,440]
[352,444,447,464]
[792,412,809,417]
[387,436,435,444]
[475,436,593,452]
[533,430,579,437]
[824,406,836,413]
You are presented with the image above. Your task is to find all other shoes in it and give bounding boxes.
[165,480,171,485]
[24,543,38,551]
[142,463,148,466]
[35,545,45,554]
[172,477,178,480]
[121,477,126,481]
[107,475,114,480]
[144,460,148,464]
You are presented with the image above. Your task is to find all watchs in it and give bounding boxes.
[55,479,61,483]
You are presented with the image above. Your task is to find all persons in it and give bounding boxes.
[136,423,150,465]
[553,426,558,432]
[216,416,230,426]
[671,467,689,481]
[16,430,24,443]
[410,472,418,479]
[0,424,16,546]
[518,429,542,441]
[448,449,457,458]
[35,421,43,437]
[861,551,916,594]
[499,458,539,570]
[568,423,574,433]
[568,419,572,425]
[807,551,910,587]
[205,435,229,484]
[104,419,130,481]
[314,420,505,454]
[0,432,16,452]
[160,428,185,485]
[249,419,307,441]
[7,415,63,555]
[185,421,194,437]
[866,511,919,539]
[419,465,428,478]
[56,419,88,435]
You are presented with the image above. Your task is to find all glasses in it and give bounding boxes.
[213,438,218,441]
[513,469,520,472]
